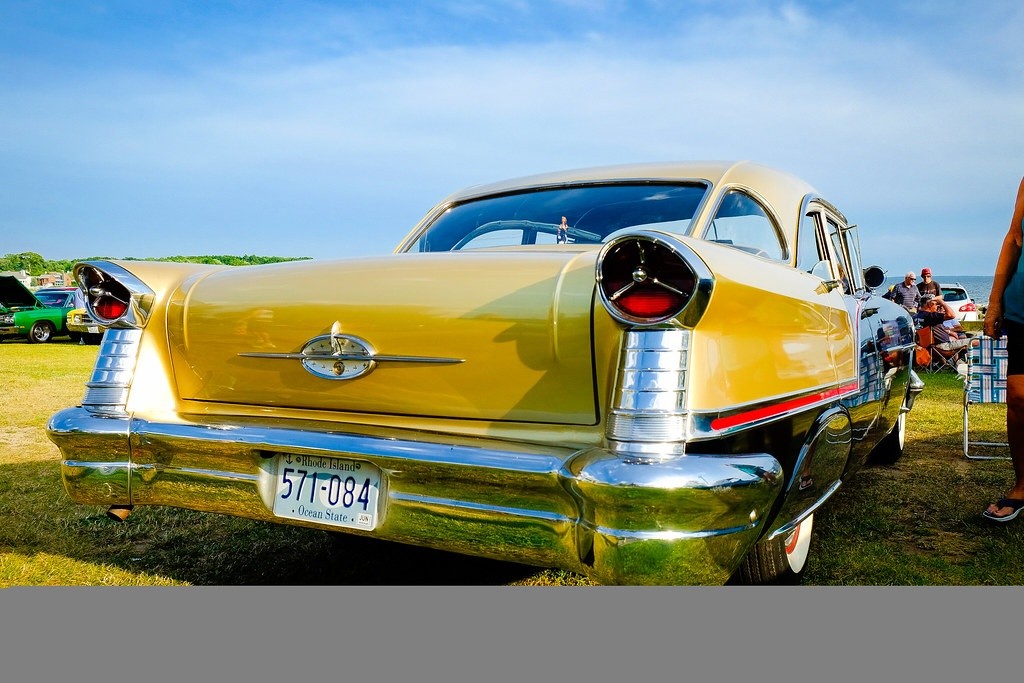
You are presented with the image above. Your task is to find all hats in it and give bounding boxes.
[921,268,932,275]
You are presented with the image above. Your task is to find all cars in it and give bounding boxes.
[46,154,925,586]
[938,281,978,323]
[0,275,84,344]
[66,306,106,346]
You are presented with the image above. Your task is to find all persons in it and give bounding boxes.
[983,176,1024,523]
[888,267,979,371]
[556,215,569,244]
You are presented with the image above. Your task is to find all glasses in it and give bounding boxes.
[910,278,917,281]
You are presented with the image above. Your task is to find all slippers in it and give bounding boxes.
[983,499,1024,522]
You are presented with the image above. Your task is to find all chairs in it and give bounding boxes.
[918,316,967,375]
[962,333,1012,460]
